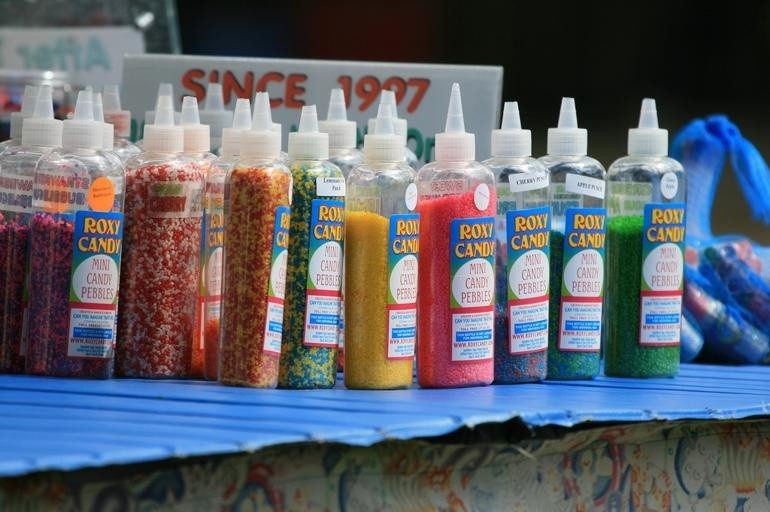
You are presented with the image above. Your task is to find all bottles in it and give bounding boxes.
[0,83,688,389]
